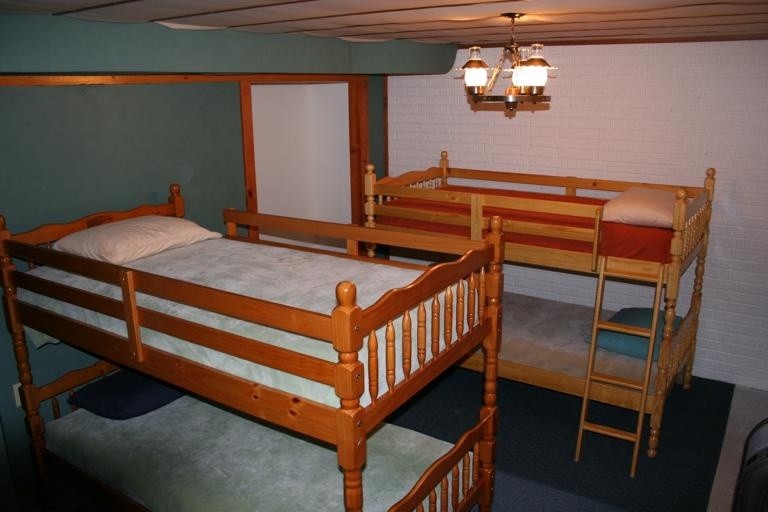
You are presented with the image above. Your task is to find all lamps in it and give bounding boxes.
[455,13,558,111]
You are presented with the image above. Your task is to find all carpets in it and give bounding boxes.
[382,365,735,512]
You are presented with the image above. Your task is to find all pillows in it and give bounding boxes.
[67,368,184,421]
[585,307,682,362]
[602,184,692,230]
[54,215,223,266]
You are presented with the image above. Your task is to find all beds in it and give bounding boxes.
[364,151,715,478]
[0,184,504,512]
